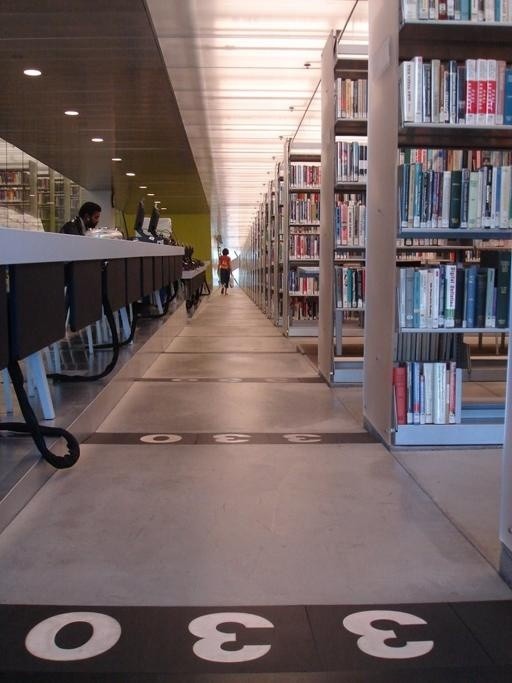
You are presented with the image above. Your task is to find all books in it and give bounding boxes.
[393,1,510,427]
[0,168,79,231]
[334,76,367,319]
[289,165,322,321]
[260,179,284,317]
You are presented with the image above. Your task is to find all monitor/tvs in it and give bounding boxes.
[134,202,154,242]
[148,207,170,245]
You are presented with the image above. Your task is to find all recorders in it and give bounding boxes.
[84,227,123,240]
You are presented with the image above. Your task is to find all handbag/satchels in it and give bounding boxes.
[230,273,234,288]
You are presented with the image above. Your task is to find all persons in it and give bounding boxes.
[217,247,233,294]
[59,201,102,236]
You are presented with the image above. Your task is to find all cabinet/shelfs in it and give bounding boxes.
[319,31,512,386]
[1,159,37,218]
[239,161,282,327]
[70,183,83,221]
[281,137,512,336]
[362,0,512,448]
[53,176,69,232]
[34,168,54,232]
